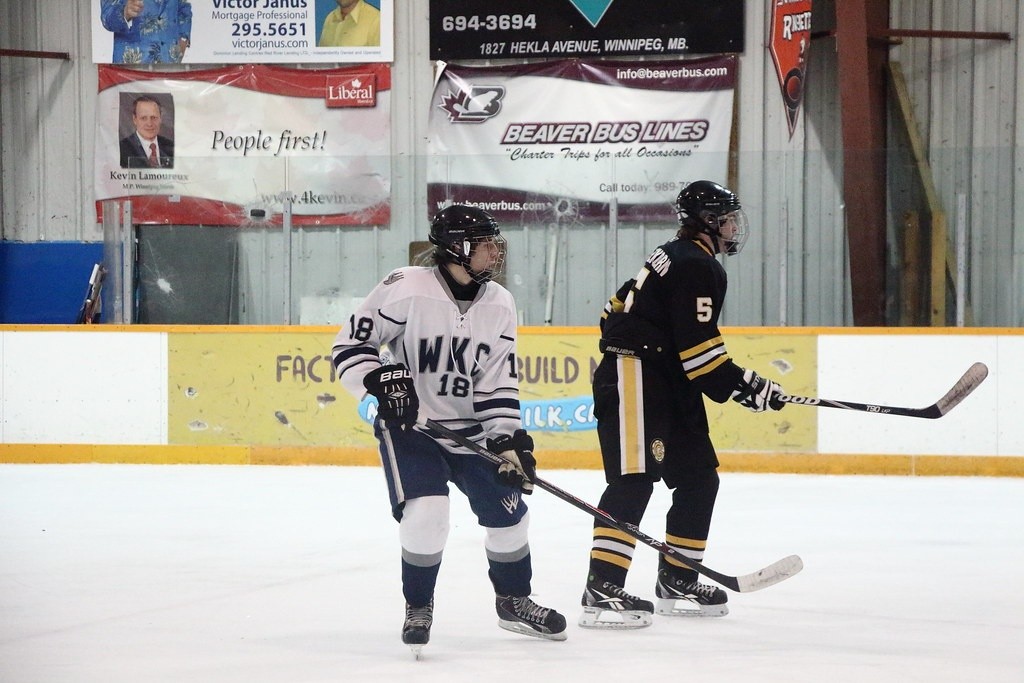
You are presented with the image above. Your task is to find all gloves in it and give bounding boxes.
[362,362,420,432]
[730,367,786,414]
[485,429,537,495]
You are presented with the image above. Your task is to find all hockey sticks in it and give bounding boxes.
[416,411,803,594]
[773,360,989,419]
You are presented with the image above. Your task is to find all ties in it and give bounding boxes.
[149,143,159,167]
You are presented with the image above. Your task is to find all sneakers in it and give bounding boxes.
[402,592,435,660]
[578,550,654,630]
[655,541,729,617]
[488,569,569,642]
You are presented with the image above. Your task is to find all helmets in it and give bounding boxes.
[676,180,742,237]
[428,204,500,267]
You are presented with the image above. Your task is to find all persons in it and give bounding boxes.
[100,0,193,64]
[581,178,787,610]
[331,203,567,643]
[119,96,174,169]
[318,0,380,46]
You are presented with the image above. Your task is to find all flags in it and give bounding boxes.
[769,1,812,142]
